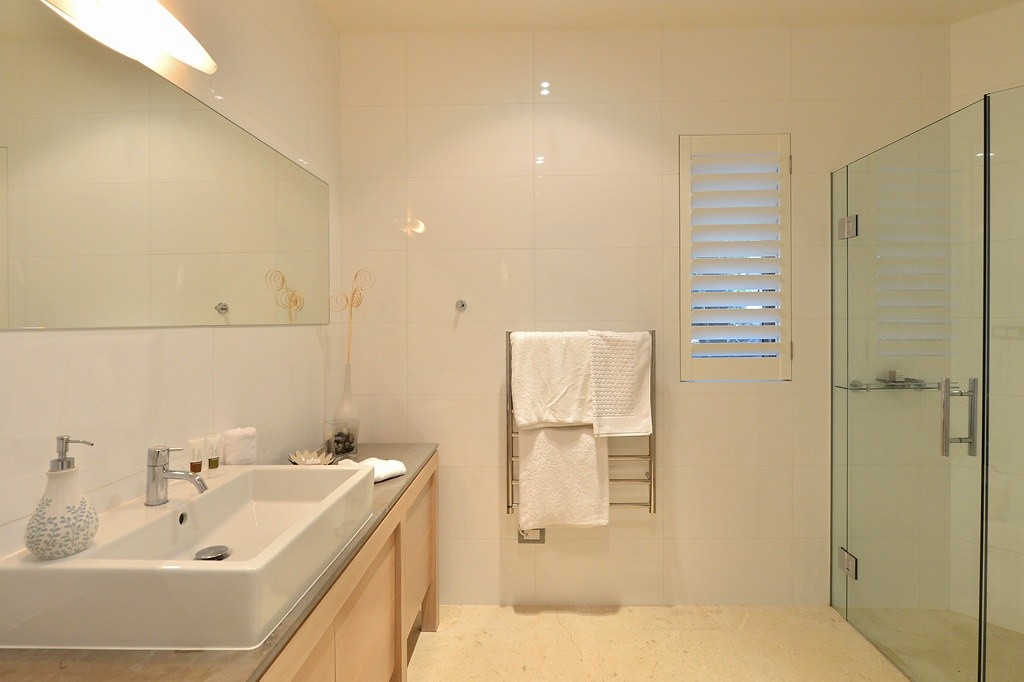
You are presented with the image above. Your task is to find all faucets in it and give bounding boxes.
[144,446,208,506]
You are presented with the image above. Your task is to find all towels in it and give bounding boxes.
[337,456,406,482]
[219,427,257,465]
[510,328,652,530]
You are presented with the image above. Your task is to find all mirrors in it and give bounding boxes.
[0,0,330,333]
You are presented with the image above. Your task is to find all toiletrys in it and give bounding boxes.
[187,438,205,474]
[206,433,222,479]
[24,434,100,560]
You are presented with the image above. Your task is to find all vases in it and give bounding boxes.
[323,417,360,458]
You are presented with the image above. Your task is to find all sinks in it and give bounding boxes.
[1,464,374,650]
